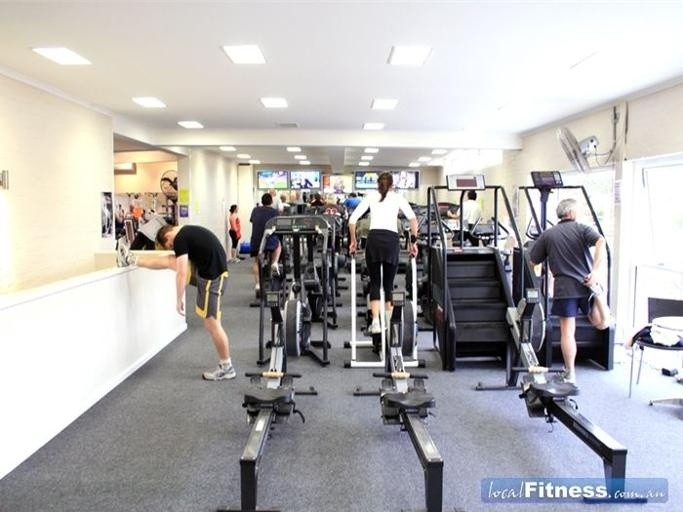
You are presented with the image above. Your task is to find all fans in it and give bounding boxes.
[160,170,178,203]
[556,123,616,174]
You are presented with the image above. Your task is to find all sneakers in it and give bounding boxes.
[271,262,280,276]
[117,240,131,268]
[582,273,606,295]
[230,258,240,262]
[552,372,575,385]
[368,317,389,333]
[255,283,260,290]
[203,362,235,380]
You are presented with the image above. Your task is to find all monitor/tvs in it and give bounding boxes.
[290,170,321,190]
[354,170,420,190]
[256,170,290,190]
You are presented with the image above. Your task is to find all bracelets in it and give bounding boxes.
[409,233,416,244]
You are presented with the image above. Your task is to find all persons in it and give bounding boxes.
[227,204,244,263]
[116,223,236,381]
[347,172,420,336]
[249,188,365,290]
[448,191,480,245]
[530,197,613,385]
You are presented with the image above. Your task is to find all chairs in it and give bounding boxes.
[626,296,683,400]
